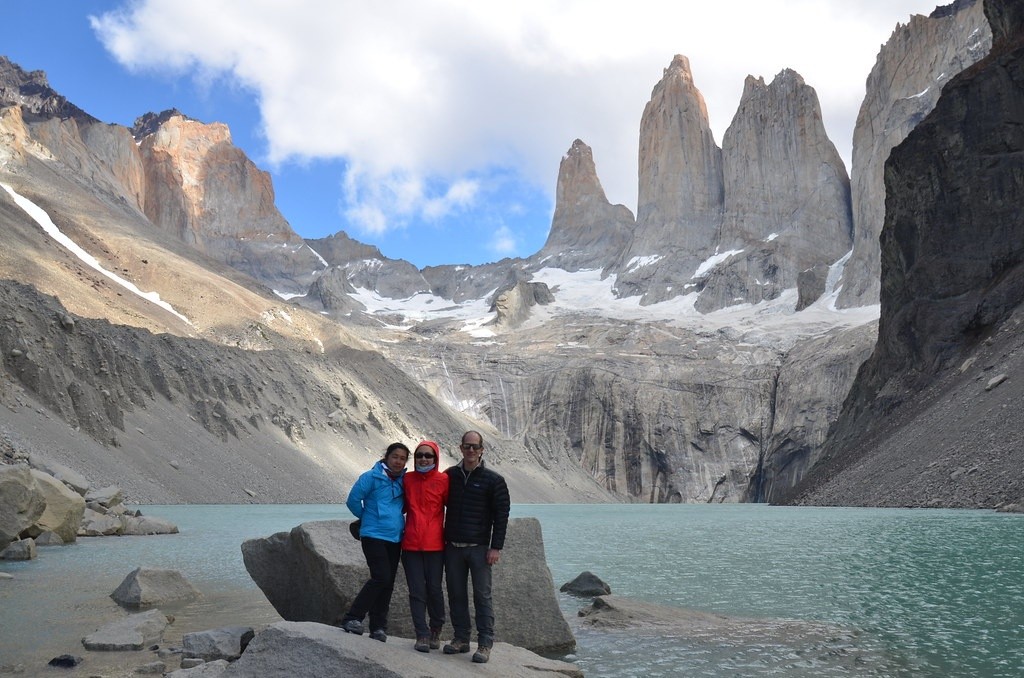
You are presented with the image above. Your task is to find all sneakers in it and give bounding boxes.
[443,639,470,654]
[473,646,491,663]
[429,632,441,649]
[369,630,387,643]
[414,638,430,653]
[344,620,363,635]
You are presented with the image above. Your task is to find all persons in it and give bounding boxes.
[441,431,511,663]
[343,443,409,642]
[400,440,452,652]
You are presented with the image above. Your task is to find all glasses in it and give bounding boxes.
[415,452,434,459]
[392,482,404,499]
[462,443,481,450]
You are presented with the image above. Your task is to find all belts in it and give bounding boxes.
[452,542,478,547]
